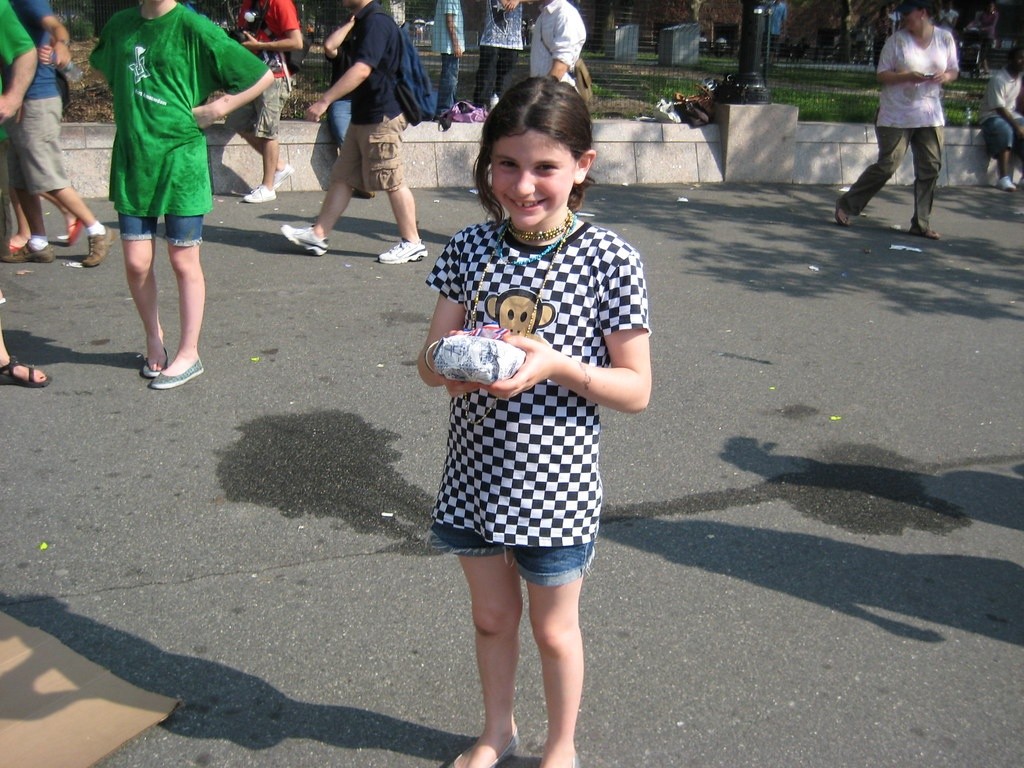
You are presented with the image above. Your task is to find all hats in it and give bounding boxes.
[893,0,938,15]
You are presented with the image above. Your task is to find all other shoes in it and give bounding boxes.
[449,726,520,768]
[66,217,82,246]
[143,347,168,378]
[353,188,375,198]
[909,226,940,240]
[8,239,27,253]
[151,356,204,389]
[835,198,849,226]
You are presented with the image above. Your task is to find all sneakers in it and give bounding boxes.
[273,163,294,189]
[280,224,330,256]
[3,239,55,263]
[996,175,1016,191]
[378,237,428,265]
[243,184,277,202]
[82,225,116,267]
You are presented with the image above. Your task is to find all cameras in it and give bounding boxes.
[228,10,260,44]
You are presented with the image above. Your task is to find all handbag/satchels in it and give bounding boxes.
[287,31,312,74]
[654,83,714,128]
[574,57,593,105]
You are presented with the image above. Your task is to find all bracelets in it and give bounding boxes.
[56,39,70,48]
[424,340,439,375]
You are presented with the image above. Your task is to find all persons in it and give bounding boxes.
[89,0,275,389]
[475,0,523,112]
[283,0,430,264]
[978,43,1024,191]
[866,2,1002,74]
[235,0,304,204]
[430,0,466,118]
[529,0,587,215]
[416,77,650,768]
[0,0,118,391]
[834,0,959,241]
[764,1,787,65]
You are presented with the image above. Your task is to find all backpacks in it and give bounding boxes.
[362,9,438,126]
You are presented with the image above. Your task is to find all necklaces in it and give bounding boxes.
[464,206,577,425]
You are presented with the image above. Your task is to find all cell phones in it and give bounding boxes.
[923,73,934,80]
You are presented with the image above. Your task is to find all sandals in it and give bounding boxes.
[0,355,52,387]
